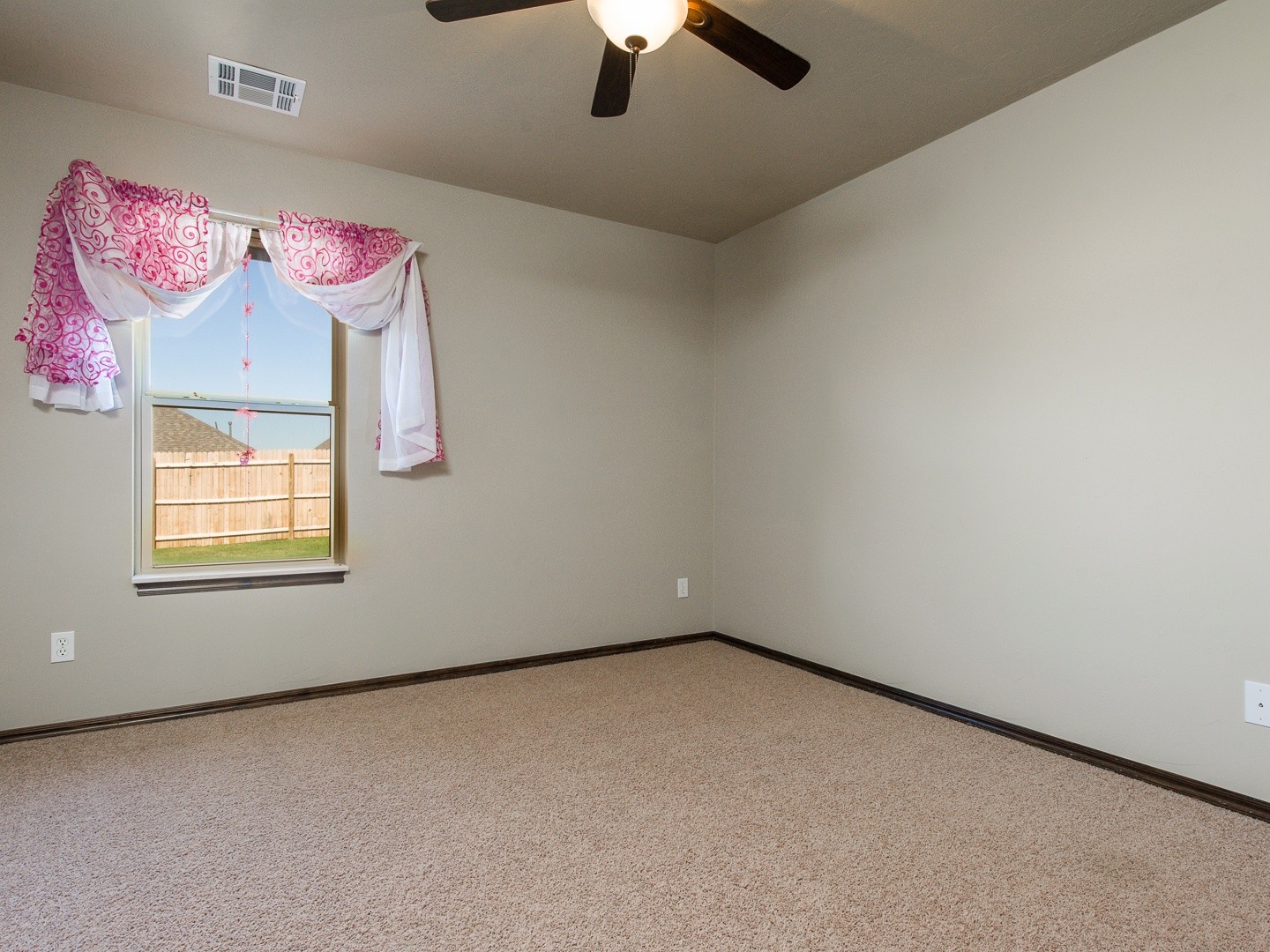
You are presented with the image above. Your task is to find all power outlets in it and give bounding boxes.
[677,578,689,598]
[51,631,75,664]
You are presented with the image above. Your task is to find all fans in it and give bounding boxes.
[424,0,811,118]
